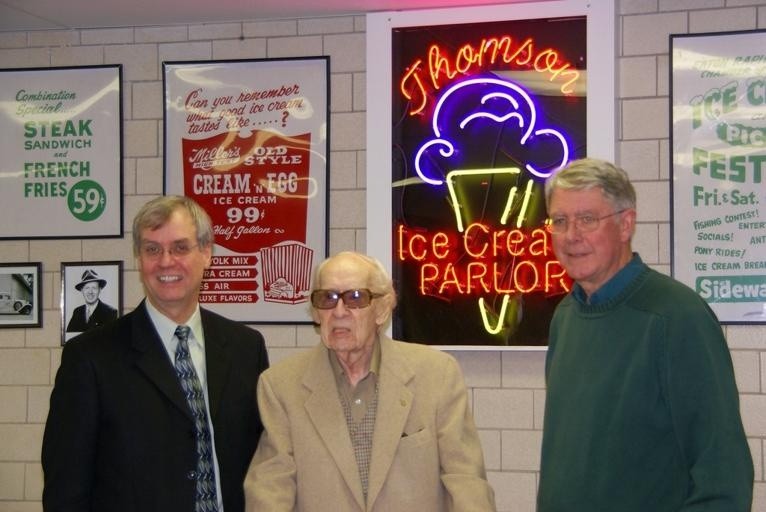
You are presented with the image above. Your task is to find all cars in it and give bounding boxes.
[0,291,33,315]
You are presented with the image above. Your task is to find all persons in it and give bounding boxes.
[536,159,755,511]
[67,267,118,330]
[39,195,269,511]
[242,251,496,511]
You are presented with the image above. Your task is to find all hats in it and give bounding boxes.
[75,269,108,291]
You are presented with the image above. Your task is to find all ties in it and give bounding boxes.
[174,324,219,512]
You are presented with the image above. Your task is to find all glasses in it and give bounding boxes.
[311,288,384,309]
[138,244,198,258]
[546,210,625,234]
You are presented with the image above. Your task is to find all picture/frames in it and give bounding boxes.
[60,260,125,346]
[1,262,43,329]
